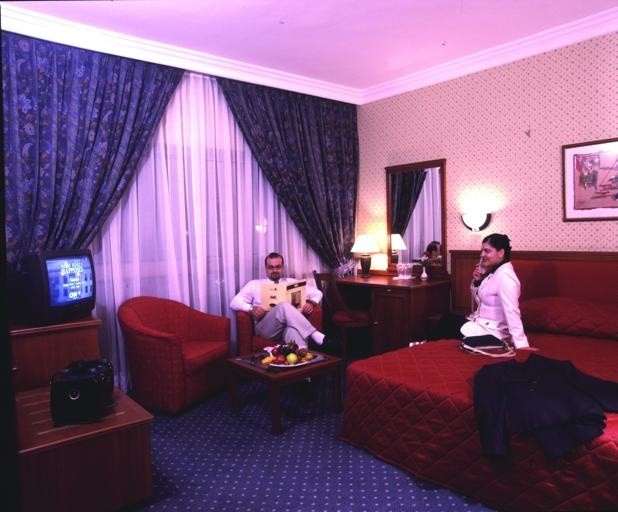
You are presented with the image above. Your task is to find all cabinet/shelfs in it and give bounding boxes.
[8,318,100,389]
[16,383,152,512]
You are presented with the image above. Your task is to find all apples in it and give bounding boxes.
[275,355,285,364]
[272,347,278,355]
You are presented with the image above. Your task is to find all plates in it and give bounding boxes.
[268,359,309,368]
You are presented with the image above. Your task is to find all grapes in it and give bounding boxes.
[278,346,293,355]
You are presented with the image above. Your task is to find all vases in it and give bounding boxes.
[421,265,427,281]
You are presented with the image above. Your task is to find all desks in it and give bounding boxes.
[335,273,452,351]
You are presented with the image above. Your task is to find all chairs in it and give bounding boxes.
[312,270,373,357]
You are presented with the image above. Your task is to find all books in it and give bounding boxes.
[259,281,307,313]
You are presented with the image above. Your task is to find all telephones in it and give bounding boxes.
[478,260,490,276]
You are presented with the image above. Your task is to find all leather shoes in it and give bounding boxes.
[308,336,339,356]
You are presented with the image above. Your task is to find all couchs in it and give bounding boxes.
[237,299,324,352]
[117,294,231,413]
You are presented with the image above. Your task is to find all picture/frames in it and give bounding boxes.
[561,137,618,223]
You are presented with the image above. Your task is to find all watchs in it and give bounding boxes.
[250,306,254,314]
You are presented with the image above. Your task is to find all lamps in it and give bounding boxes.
[461,213,492,232]
[350,233,385,278]
[390,233,408,269]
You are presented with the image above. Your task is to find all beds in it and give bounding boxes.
[341,249,618,511]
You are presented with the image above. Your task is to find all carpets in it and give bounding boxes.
[147,370,488,510]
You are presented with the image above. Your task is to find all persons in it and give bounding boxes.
[422,233,539,351]
[423,240,441,260]
[229,253,341,353]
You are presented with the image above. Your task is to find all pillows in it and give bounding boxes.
[518,297,618,338]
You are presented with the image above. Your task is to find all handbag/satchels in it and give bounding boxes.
[459,334,517,358]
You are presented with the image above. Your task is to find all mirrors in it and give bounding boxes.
[384,158,447,276]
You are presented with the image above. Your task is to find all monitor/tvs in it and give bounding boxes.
[14,249,96,325]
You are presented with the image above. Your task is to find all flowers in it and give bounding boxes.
[419,254,429,265]
